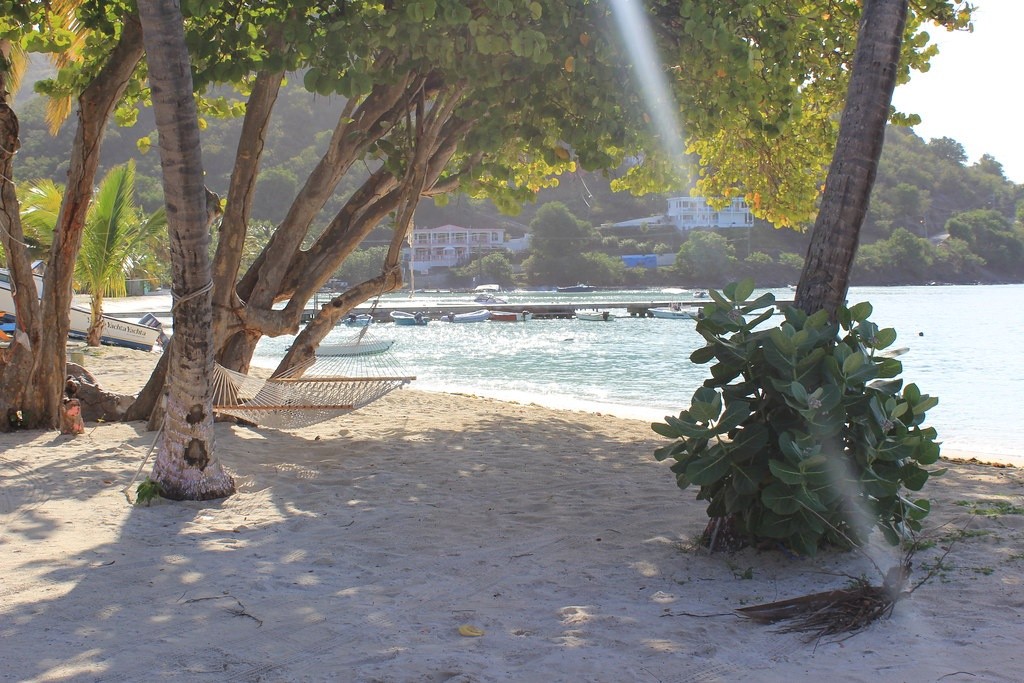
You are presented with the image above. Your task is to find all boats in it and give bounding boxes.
[575,310,616,321]
[1,260,161,353]
[555,284,595,291]
[440,309,490,323]
[473,284,509,307]
[648,294,696,319]
[389,311,430,325]
[345,313,373,327]
[694,289,705,298]
[490,309,534,321]
